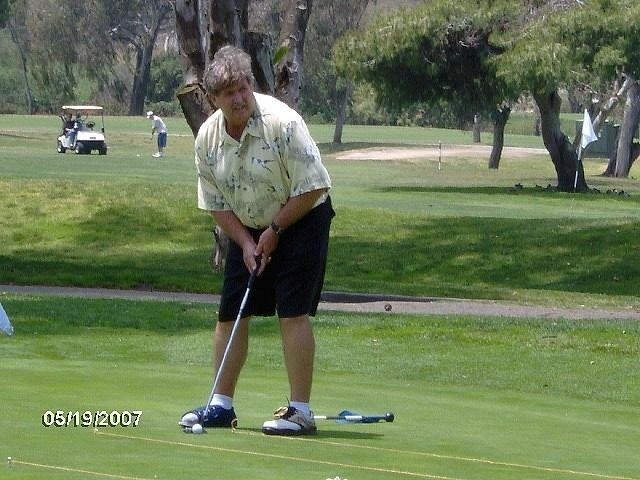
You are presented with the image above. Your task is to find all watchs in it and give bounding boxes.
[270,221,285,237]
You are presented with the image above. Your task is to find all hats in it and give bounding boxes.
[145,110,153,119]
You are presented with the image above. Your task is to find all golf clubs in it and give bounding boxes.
[177,254,260,428]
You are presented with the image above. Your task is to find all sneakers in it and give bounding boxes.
[152,152,165,158]
[260,405,321,437]
[176,405,239,430]
[69,143,74,148]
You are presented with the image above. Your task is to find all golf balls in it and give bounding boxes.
[192,424,204,434]
[136,153,141,157]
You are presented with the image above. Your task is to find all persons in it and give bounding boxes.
[146,110,168,158]
[179,46,336,435]
[63,112,82,147]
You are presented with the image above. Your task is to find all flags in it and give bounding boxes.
[581,108,598,148]
